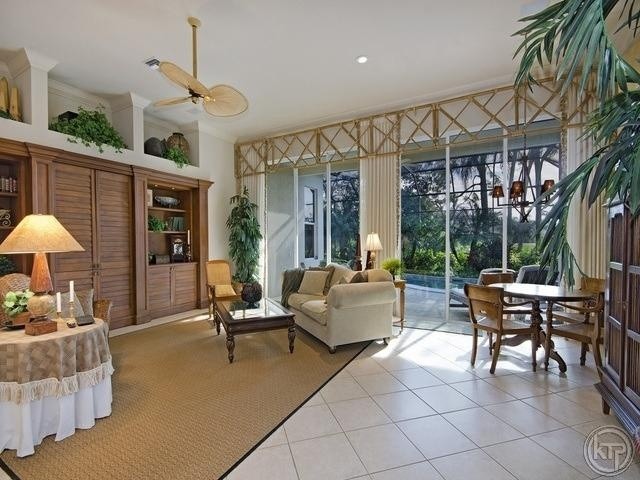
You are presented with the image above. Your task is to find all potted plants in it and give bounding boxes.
[223,183,264,302]
[2,289,36,329]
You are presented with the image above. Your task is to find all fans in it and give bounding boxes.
[150,17,250,119]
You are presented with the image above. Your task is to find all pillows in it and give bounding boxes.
[51,290,85,317]
[294,264,369,307]
[74,287,95,317]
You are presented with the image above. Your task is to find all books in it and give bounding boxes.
[0,176,19,194]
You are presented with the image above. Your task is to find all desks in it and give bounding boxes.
[391,277,408,333]
[1,315,116,460]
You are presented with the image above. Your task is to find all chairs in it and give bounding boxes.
[516,264,553,286]
[448,262,517,312]
[204,259,246,318]
[544,289,609,379]
[553,274,608,368]
[462,280,540,376]
[0,271,113,349]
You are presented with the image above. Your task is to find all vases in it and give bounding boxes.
[167,130,191,158]
[144,137,169,158]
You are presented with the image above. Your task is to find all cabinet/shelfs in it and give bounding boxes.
[0,136,27,279]
[142,262,200,319]
[132,164,214,266]
[29,145,144,328]
[594,187,640,442]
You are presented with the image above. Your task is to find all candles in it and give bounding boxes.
[69,280,75,303]
[55,291,61,313]
[187,227,191,245]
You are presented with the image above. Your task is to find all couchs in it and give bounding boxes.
[279,260,397,355]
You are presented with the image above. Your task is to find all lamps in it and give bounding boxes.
[0,212,87,337]
[364,230,382,269]
[491,132,555,226]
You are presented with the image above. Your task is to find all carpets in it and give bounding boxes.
[1,313,376,479]
